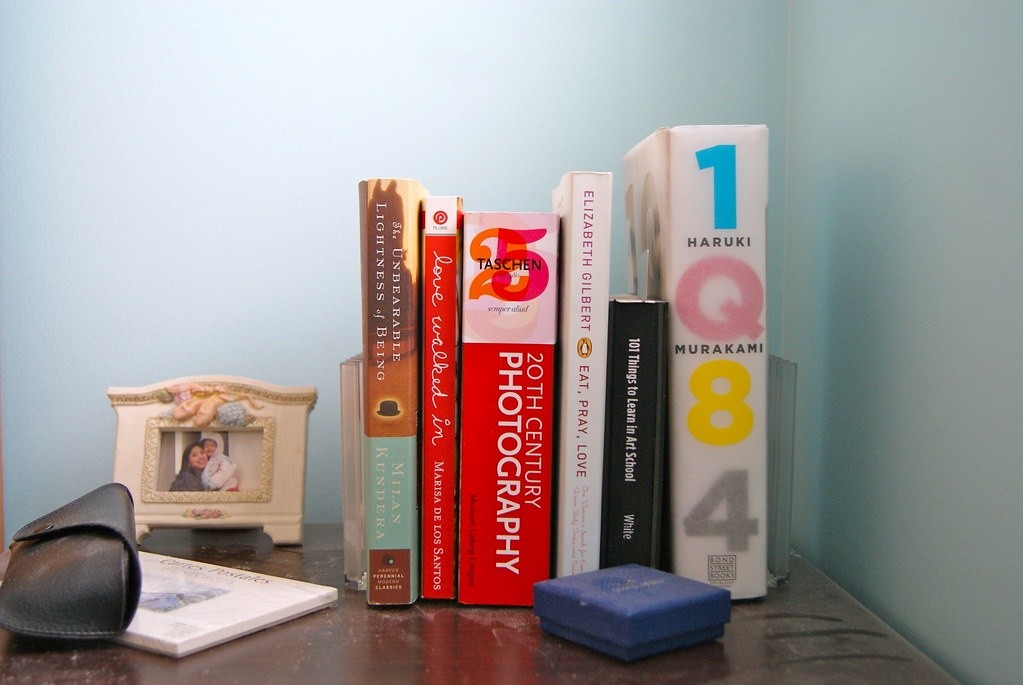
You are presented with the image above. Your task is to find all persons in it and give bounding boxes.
[169,431,239,492]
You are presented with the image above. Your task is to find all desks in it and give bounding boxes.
[0,521,964,685]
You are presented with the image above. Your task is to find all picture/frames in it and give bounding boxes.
[105,375,319,548]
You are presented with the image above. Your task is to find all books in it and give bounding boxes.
[118,549,339,657]
[337,121,770,604]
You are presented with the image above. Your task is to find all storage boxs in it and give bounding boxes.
[530,562,735,663]
